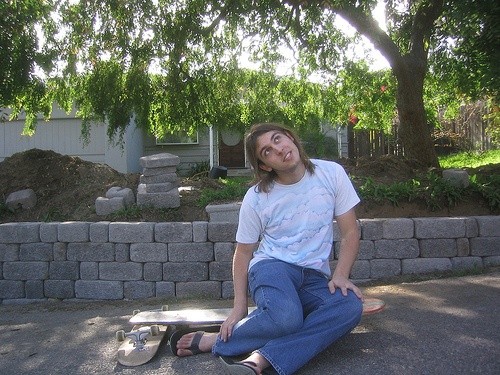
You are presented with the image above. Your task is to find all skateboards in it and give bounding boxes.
[115,305,172,367]
[129,296,386,332]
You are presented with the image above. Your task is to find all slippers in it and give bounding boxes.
[170,325,222,357]
[219,356,262,375]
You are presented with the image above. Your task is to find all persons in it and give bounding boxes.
[170,124,363,374]
